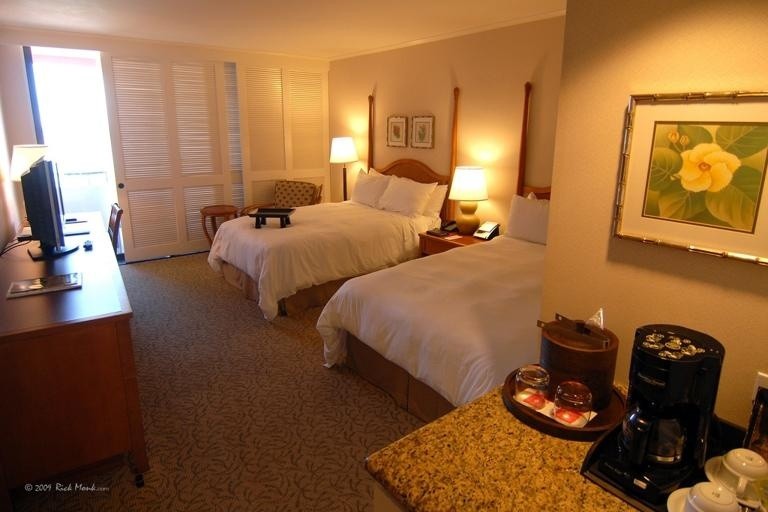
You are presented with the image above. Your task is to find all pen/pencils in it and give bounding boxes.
[66,218,77,221]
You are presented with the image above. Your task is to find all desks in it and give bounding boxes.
[364,365,661,512]
[0,209,152,486]
[200,204,239,247]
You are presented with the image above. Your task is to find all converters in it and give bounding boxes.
[17,235,32,242]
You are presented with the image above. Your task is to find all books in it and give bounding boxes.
[4,272,82,299]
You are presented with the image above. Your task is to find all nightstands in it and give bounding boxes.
[417,227,477,258]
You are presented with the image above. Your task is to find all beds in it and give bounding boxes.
[205,84,460,317]
[315,81,548,423]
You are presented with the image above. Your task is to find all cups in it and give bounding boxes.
[666,447,767,512]
[551,380,593,428]
[514,362,550,412]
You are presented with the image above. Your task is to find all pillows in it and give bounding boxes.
[368,167,385,176]
[378,174,437,218]
[505,193,548,247]
[400,177,447,219]
[352,167,391,208]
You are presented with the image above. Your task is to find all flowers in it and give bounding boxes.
[647,123,768,232]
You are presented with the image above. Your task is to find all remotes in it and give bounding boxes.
[84,239,93,248]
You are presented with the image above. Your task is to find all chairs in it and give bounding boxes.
[106,202,123,249]
[241,179,323,216]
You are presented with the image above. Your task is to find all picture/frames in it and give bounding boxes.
[411,116,434,149]
[386,115,407,147]
[607,89,768,278]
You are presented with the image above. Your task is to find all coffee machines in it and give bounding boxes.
[580,323,727,512]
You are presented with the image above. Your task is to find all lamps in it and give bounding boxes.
[329,136,358,201]
[448,165,489,235]
[8,144,56,183]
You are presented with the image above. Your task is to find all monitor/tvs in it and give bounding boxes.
[20,157,78,259]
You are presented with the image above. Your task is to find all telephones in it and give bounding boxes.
[440,220,457,232]
